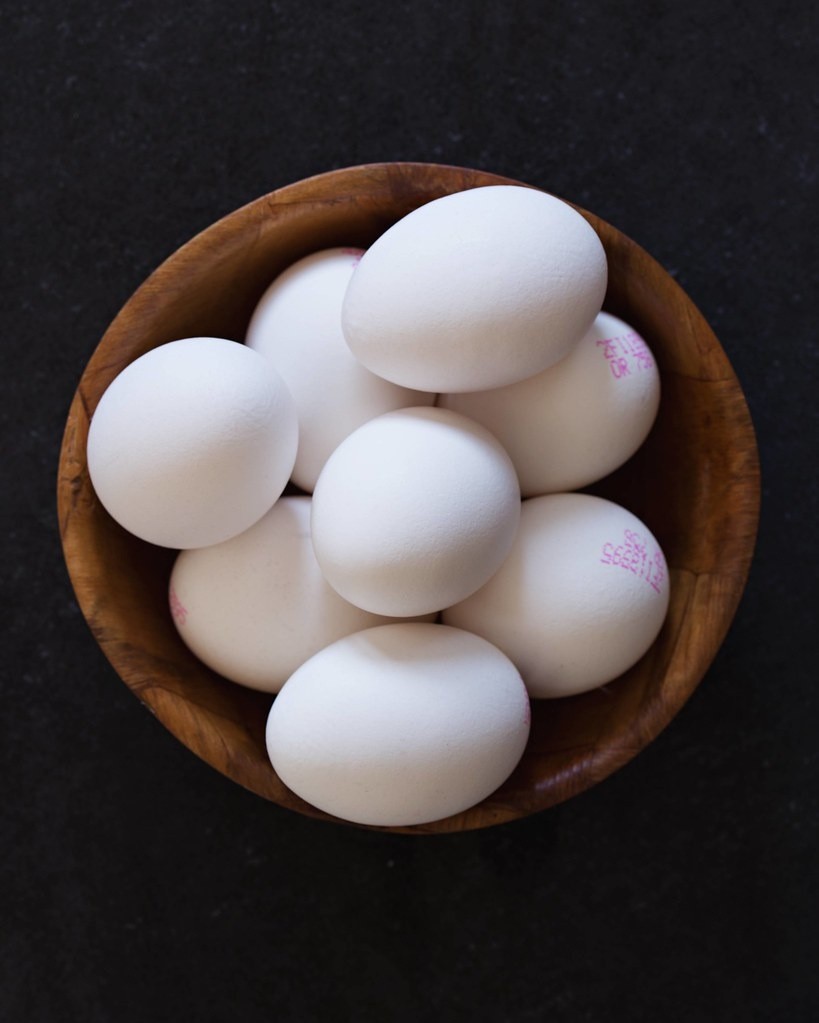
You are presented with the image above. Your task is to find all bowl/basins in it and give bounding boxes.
[57,160,760,829]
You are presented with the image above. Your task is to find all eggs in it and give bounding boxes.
[87,185,668,829]
